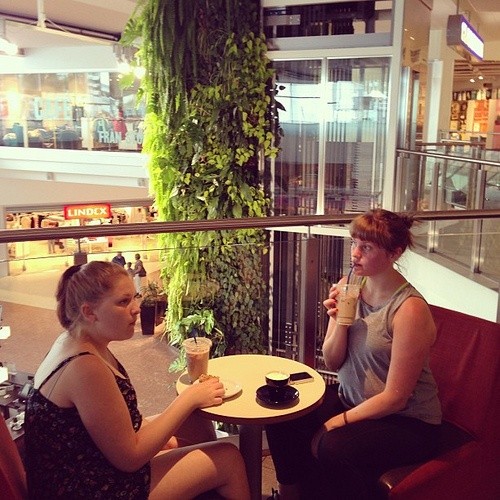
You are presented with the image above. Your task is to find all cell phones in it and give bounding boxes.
[289,371,313,385]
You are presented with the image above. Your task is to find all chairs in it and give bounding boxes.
[375,305,500,500]
[0,417,26,500]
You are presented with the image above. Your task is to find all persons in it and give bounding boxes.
[23,260,251,500]
[263,208,442,500]
[110,109,128,140]
[111,248,147,299]
[7,208,128,250]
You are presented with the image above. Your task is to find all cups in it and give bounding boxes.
[264,370,290,401]
[332,283,361,326]
[182,337,212,384]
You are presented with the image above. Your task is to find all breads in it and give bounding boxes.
[199,374,220,382]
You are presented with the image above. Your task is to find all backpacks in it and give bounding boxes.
[139,267,146,277]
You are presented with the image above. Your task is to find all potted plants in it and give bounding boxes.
[138,281,159,335]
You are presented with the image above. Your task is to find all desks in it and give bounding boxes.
[0,367,39,414]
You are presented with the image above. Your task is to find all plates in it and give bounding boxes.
[193,377,242,399]
[256,385,299,406]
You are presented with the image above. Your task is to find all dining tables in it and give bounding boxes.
[176,355,326,500]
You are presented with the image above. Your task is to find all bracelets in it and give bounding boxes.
[343,411,349,424]
